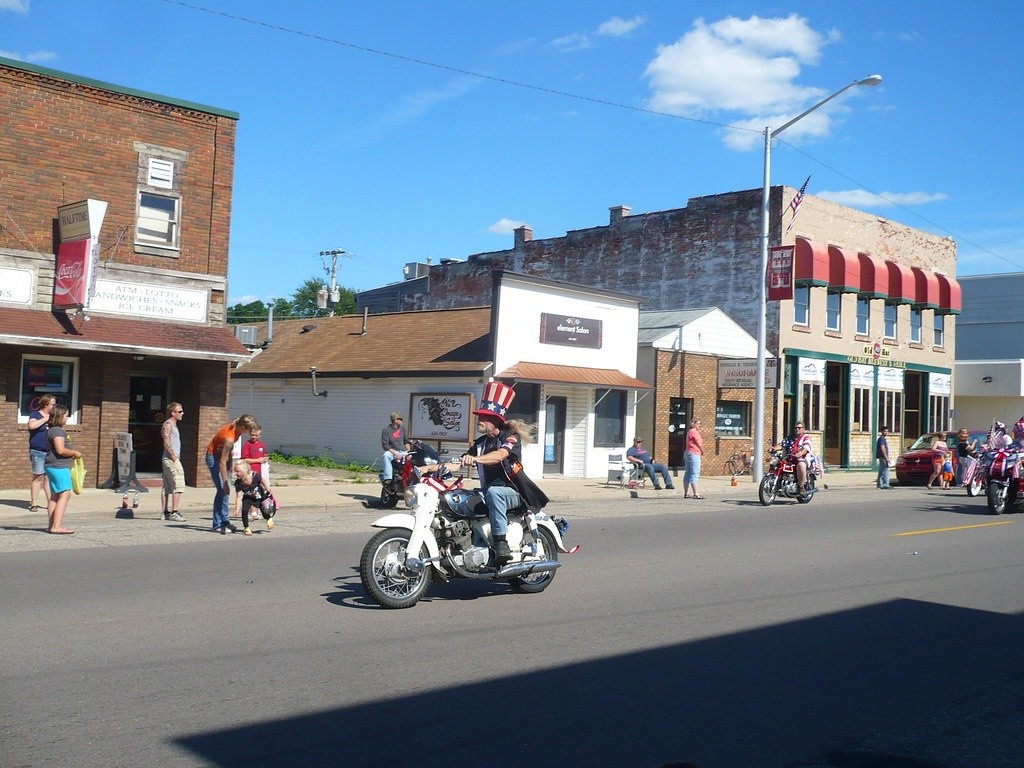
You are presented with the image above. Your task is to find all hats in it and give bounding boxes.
[472,382,516,421]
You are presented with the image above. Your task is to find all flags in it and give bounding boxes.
[786,176,812,234]
[961,454,979,487]
[986,423,1003,450]
[815,452,824,478]
[1012,450,1022,479]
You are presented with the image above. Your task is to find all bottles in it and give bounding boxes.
[732,474,738,486]
[123,494,129,508]
[133,494,138,508]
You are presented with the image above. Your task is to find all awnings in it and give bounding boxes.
[493,360,656,409]
[794,237,962,316]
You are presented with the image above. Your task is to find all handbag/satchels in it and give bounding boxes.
[934,451,942,457]
[71,455,88,495]
[261,460,270,488]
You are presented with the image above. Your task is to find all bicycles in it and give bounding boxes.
[723,445,744,475]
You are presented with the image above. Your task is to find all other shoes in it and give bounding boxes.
[800,487,806,494]
[878,486,893,489]
[941,487,951,490]
[494,540,513,560]
[655,485,662,490]
[215,514,274,536]
[665,484,674,489]
[384,479,392,484]
[927,485,933,490]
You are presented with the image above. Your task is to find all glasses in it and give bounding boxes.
[636,440,642,443]
[172,410,183,414]
[395,418,402,421]
[795,427,803,429]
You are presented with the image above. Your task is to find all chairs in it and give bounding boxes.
[606,455,651,489]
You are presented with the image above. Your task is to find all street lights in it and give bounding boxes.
[752,74,885,483]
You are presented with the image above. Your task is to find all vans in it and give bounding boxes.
[896,430,991,486]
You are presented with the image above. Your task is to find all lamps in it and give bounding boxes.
[983,377,993,383]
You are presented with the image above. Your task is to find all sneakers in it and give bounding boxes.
[161,511,171,520]
[169,513,187,522]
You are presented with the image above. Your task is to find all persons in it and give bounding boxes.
[233,459,274,536]
[242,424,269,522]
[991,420,1014,446]
[159,402,186,523]
[926,432,955,490]
[204,415,257,534]
[381,412,411,485]
[876,426,894,489]
[956,428,974,486]
[683,418,706,500]
[626,436,675,490]
[44,405,86,534]
[28,394,56,511]
[767,421,812,494]
[416,382,550,561]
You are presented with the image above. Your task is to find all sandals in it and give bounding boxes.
[693,494,703,499]
[684,494,691,498]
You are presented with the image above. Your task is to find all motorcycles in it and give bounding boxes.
[378,439,449,508]
[758,450,819,506]
[966,443,1024,514]
[360,457,580,609]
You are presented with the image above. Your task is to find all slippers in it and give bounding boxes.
[30,505,38,512]
[48,527,75,534]
